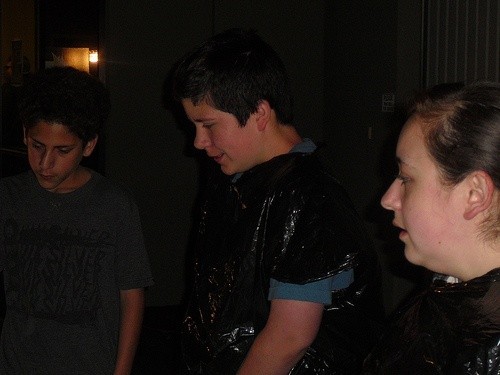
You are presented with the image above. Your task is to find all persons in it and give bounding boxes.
[0,66,154,375]
[164,28,500,375]
